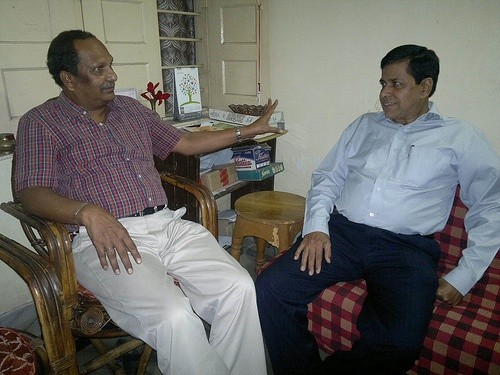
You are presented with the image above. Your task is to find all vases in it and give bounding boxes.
[149,100,157,112]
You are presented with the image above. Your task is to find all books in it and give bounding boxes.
[200,165,239,193]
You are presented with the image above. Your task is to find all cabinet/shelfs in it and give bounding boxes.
[154,120,277,223]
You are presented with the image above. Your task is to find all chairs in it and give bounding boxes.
[0,170,219,375]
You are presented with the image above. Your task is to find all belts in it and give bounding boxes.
[126,203,165,217]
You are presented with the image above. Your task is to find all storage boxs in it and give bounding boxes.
[201,145,285,190]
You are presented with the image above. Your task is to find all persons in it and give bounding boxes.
[11,29,267,375]
[254,45,500,375]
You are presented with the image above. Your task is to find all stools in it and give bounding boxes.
[232,191,305,261]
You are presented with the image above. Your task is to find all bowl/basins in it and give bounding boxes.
[0,133,16,155]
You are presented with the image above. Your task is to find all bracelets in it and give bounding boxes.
[73,202,90,225]
[233,126,243,143]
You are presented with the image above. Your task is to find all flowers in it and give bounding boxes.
[141,81,170,106]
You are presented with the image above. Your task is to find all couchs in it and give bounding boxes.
[257,184,500,375]
